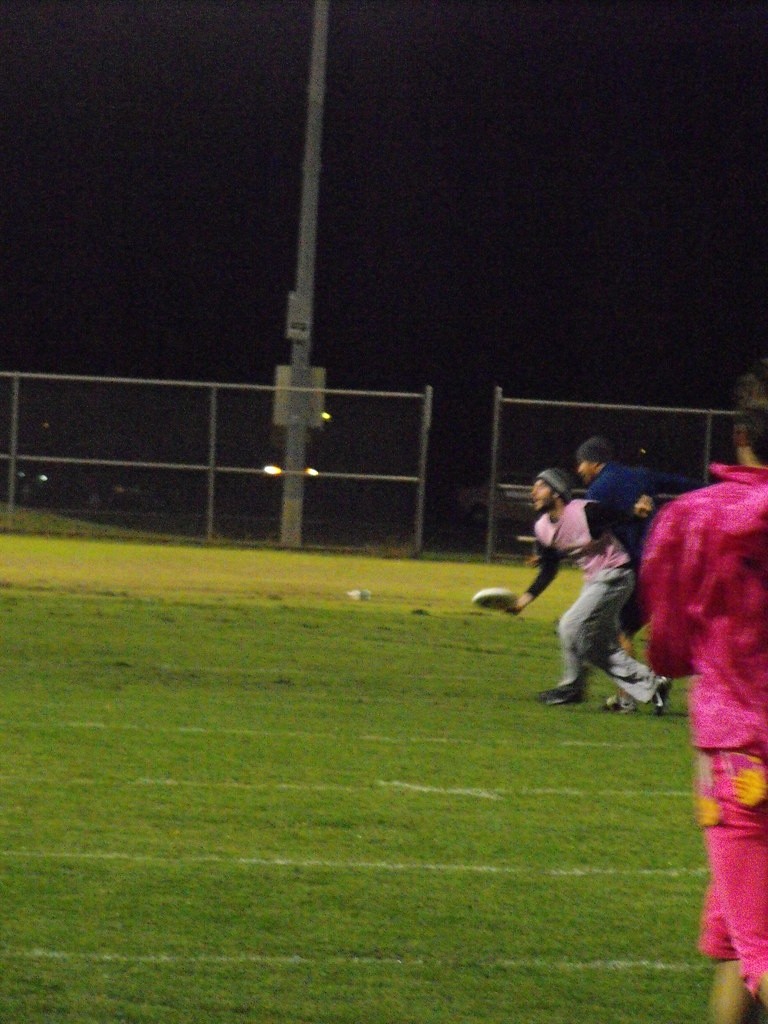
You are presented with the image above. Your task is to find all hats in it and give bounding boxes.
[535,467,573,501]
[577,436,609,462]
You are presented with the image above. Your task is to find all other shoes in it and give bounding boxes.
[599,695,641,714]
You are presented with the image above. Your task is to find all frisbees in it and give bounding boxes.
[472,587,519,611]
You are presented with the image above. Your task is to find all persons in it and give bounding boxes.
[508,438,706,719]
[636,356,768,1024]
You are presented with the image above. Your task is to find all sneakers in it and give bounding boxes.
[652,675,673,717]
[539,684,582,707]
